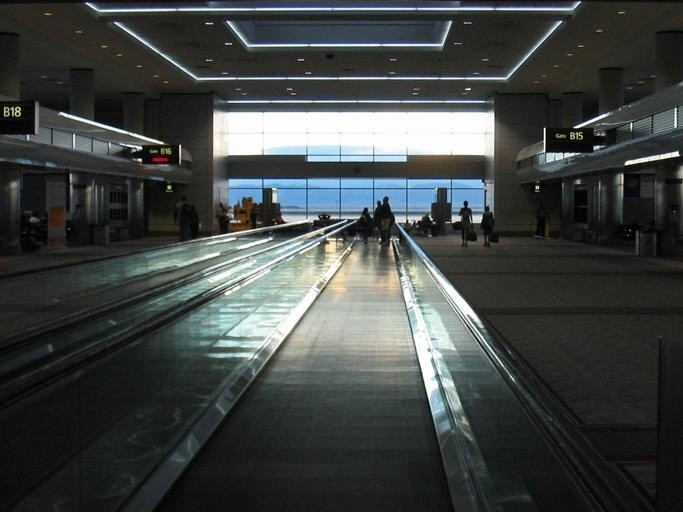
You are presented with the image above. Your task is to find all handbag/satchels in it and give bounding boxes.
[490,231,498,242]
[469,231,477,240]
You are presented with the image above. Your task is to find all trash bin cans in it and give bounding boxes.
[636,227,657,257]
[92,220,110,246]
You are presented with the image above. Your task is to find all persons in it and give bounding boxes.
[173,196,199,242]
[359,207,372,242]
[218,208,229,234]
[535,202,547,239]
[458,201,473,247]
[249,208,257,229]
[378,196,390,247]
[481,206,493,248]
[373,199,384,227]
[215,203,225,218]
[275,212,285,224]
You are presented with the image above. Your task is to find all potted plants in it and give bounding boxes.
[92,215,109,245]
[128,212,145,239]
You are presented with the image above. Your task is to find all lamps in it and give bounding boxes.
[534,176,541,193]
[164,180,174,193]
